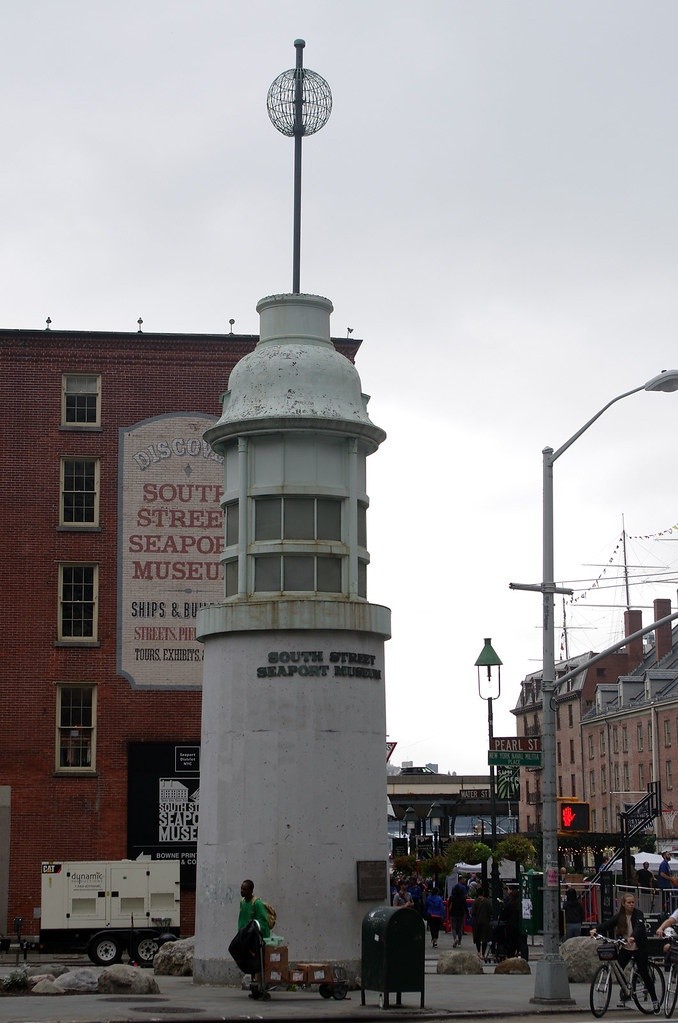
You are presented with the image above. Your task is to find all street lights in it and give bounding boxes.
[426,801,446,888]
[528,366,678,1004]
[402,806,420,858]
[474,638,504,929]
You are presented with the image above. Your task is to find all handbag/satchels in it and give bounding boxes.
[597,944,618,960]
[670,944,678,962]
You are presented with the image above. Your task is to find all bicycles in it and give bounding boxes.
[654,929,678,1018]
[589,933,666,1018]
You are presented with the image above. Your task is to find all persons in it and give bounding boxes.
[390,870,586,963]
[238,879,271,1000]
[591,852,678,1016]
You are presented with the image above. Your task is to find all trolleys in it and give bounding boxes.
[249,918,350,1002]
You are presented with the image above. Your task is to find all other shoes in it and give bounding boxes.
[478,952,480,956]
[249,992,259,999]
[481,955,486,959]
[453,940,458,948]
[433,942,438,947]
[617,1000,624,1007]
[458,942,461,947]
[652,1001,661,1015]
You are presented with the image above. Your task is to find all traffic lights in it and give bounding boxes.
[473,824,479,836]
[559,801,591,831]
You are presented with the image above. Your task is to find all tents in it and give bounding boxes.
[447,855,527,898]
[604,852,678,886]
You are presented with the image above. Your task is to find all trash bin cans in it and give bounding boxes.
[360,907,424,992]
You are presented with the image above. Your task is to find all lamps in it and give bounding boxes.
[229,319,235,334]
[137,317,143,333]
[46,317,51,331]
[347,328,353,339]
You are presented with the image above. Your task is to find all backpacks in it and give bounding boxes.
[239,896,278,930]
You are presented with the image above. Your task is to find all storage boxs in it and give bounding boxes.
[288,970,306,983]
[297,964,333,983]
[264,946,288,983]
